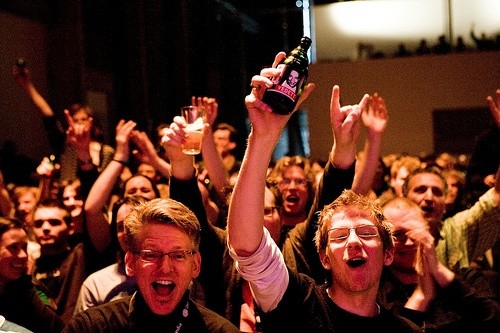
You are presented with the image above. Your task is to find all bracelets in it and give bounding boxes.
[109,158,128,166]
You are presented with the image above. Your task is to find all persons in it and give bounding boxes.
[377,197,500,333]
[227,52,421,333]
[0,65,500,333]
[62,198,239,333]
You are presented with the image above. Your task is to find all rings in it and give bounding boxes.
[249,82,262,91]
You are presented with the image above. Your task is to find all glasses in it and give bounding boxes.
[133,250,197,262]
[326,225,380,240]
[264,206,279,217]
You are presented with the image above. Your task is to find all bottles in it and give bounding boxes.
[262,36,312,116]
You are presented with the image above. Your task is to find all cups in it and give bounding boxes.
[180,106,206,155]
